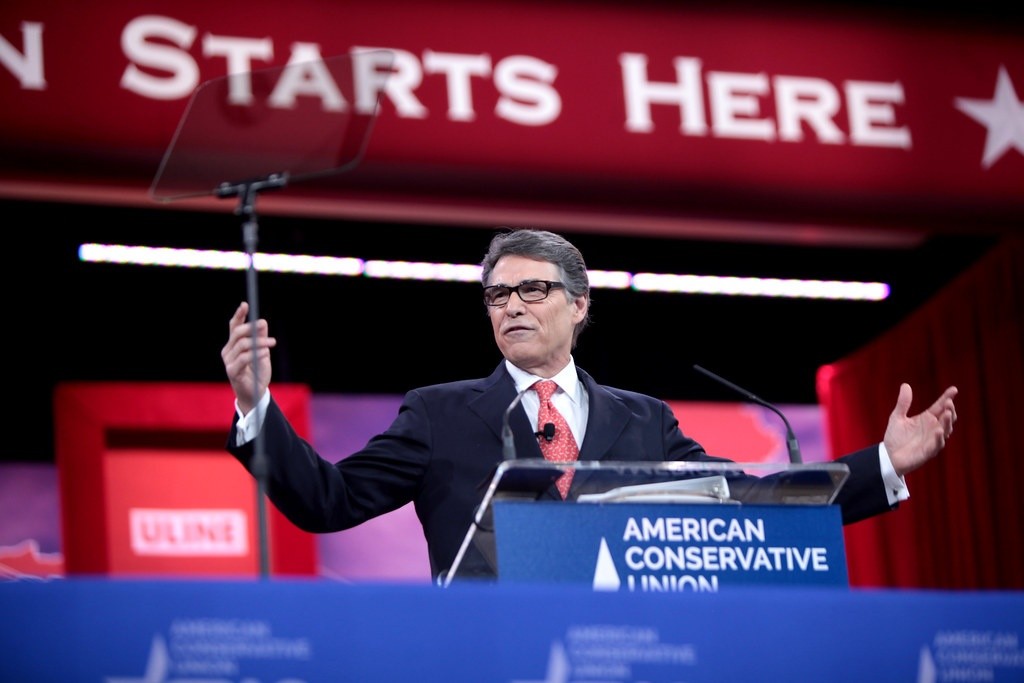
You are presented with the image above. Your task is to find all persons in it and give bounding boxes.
[219,229,959,586]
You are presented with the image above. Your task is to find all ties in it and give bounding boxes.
[528,380,579,500]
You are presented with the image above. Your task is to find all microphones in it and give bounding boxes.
[695,364,835,505]
[543,423,555,440]
[481,390,565,503]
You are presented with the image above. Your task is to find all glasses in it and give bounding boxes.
[482,280,565,306]
